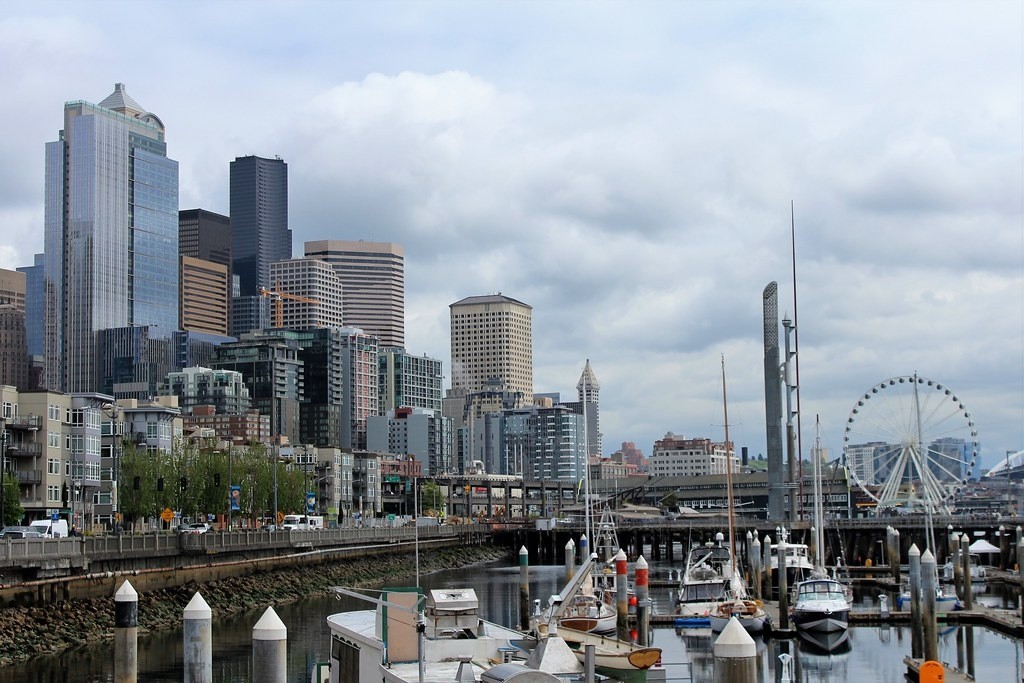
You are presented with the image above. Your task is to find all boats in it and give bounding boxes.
[941,583,987,609]
[899,587,958,611]
[943,549,989,582]
[936,622,957,637]
[791,414,853,632]
[762,526,814,593]
[310,355,773,683]
[797,629,852,683]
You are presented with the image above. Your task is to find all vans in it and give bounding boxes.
[309,516,323,530]
[30,518,68,538]
[280,515,310,530]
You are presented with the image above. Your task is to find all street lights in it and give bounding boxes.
[346,479,363,518]
[318,475,336,517]
[273,437,279,531]
[221,433,234,533]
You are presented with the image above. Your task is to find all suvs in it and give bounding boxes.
[0,526,42,539]
[558,518,571,524]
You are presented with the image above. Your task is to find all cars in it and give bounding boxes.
[181,523,212,534]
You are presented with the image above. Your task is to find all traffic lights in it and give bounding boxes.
[181,478,187,492]
